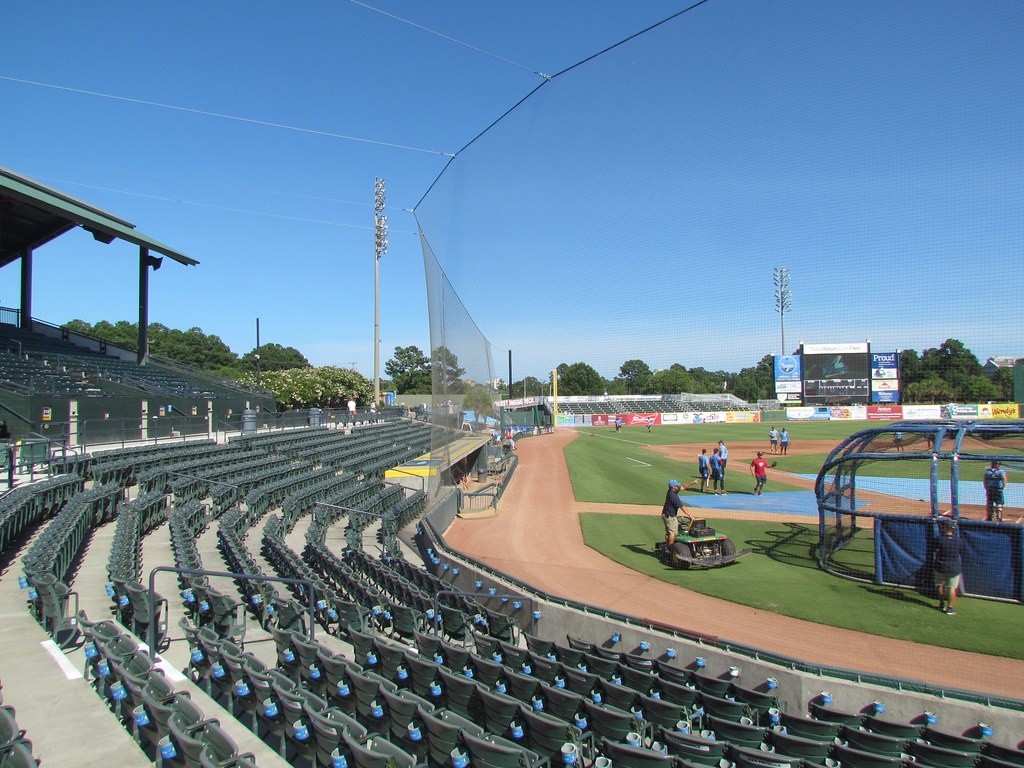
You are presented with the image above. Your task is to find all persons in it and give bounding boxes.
[778,427,790,455]
[507,428,517,450]
[750,451,777,496]
[717,440,728,480]
[347,397,358,417]
[709,448,728,496]
[924,432,934,452]
[370,400,377,414]
[932,520,964,615]
[698,448,711,493]
[983,458,1007,521]
[661,478,700,553]
[769,426,780,454]
[647,417,653,432]
[436,396,453,407]
[615,417,622,433]
[893,430,904,452]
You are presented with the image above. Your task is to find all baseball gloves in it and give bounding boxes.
[771,461,777,467]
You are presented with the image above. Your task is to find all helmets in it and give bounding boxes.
[992,460,1002,467]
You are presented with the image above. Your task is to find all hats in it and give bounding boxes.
[713,448,718,452]
[668,480,681,487]
[939,518,955,530]
[771,426,774,429]
[757,451,764,455]
[783,427,785,429]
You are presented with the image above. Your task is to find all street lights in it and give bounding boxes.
[773,267,792,356]
[373,176,389,410]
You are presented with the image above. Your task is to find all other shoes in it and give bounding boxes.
[982,517,993,522]
[758,491,761,495]
[715,492,719,495]
[721,490,728,495]
[947,607,956,615]
[754,488,757,492]
[939,604,946,611]
[666,549,672,553]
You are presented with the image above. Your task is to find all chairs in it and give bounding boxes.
[687,517,706,535]
[0,322,1024,768]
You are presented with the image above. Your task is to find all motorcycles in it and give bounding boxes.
[654,516,752,570]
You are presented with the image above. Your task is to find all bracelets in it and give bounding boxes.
[689,481,692,485]
